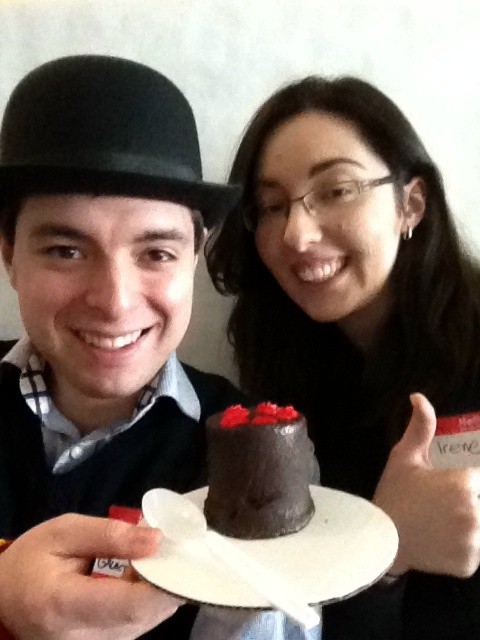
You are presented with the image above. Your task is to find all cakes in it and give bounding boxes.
[200,401,316,540]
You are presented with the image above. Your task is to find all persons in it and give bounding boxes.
[204,75,480,640]
[0,56,246,640]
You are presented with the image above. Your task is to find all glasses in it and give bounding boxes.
[240,169,405,231]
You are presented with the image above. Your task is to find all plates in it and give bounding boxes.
[131,485,400,611]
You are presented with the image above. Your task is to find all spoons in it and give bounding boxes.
[141,487,319,631]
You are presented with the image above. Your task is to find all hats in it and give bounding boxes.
[1,55,244,231]
